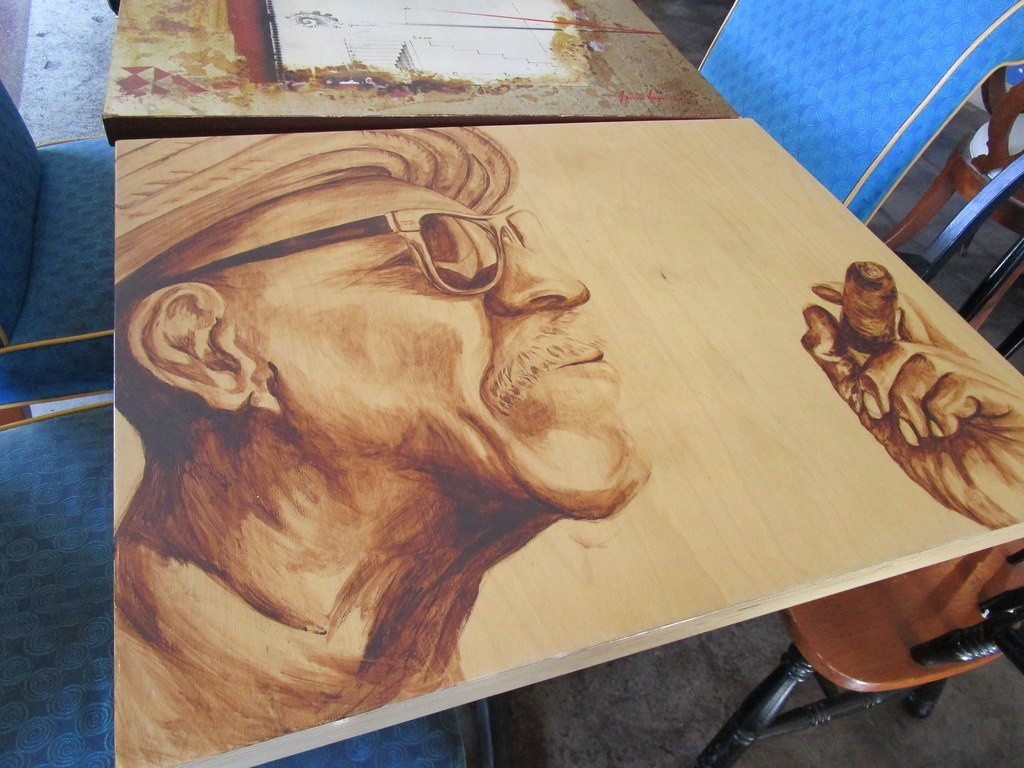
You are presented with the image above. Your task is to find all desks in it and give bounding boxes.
[112,119,1022,768]
[100,1,741,149]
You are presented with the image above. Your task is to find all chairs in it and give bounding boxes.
[1,78,116,429]
[686,228,1020,764]
[1,401,495,766]
[696,1,1023,230]
[880,66,1024,334]
